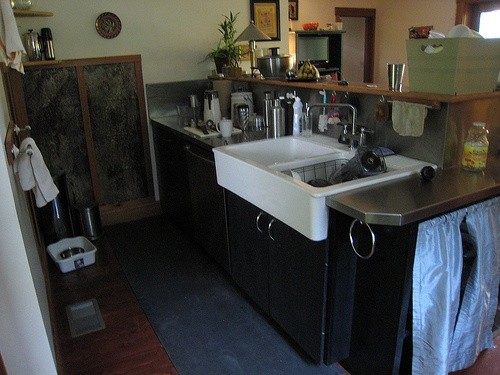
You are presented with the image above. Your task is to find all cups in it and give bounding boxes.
[387,64,406,92]
[215,119,233,137]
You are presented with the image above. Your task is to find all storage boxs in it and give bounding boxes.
[405,37,500,96]
[48,236,97,274]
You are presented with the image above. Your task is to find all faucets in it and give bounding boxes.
[306,102,358,149]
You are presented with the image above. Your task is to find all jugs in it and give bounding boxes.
[24,28,44,62]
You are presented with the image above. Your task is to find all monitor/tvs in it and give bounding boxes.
[298,36,330,66]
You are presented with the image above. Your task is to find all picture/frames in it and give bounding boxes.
[96,12,121,39]
[250,0,281,42]
[288,0,298,20]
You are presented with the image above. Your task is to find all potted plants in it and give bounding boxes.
[198,10,252,74]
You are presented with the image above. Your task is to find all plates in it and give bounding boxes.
[286,77,325,82]
[95,11,122,39]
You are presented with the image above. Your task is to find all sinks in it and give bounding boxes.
[278,154,382,242]
[211,136,343,196]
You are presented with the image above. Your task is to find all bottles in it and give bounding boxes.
[40,28,56,62]
[461,122,491,172]
[244,113,266,138]
[202,89,303,139]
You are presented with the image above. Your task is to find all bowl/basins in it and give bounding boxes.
[61,247,85,258]
[303,22,319,31]
[175,104,201,119]
[258,58,290,81]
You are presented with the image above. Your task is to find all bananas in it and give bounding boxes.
[297,59,320,79]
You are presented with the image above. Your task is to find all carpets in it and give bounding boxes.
[105,210,345,375]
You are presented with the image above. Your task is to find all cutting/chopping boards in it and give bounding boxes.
[184,125,243,138]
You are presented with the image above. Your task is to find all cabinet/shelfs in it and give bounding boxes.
[223,189,354,366]
[4,53,163,226]
[150,122,229,272]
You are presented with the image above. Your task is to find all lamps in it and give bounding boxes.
[235,21,272,75]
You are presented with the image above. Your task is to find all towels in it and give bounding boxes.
[0,0,28,75]
[11,135,60,209]
